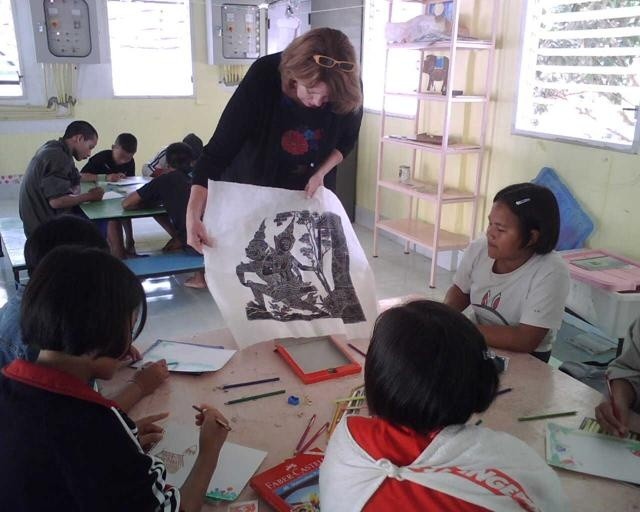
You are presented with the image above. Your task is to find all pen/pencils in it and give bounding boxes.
[222,378,280,389]
[192,405,231,431]
[519,412,578,421]
[141,362,179,370]
[605,373,627,438]
[92,180,98,189]
[294,383,368,455]
[580,418,640,442]
[498,388,513,394]
[476,420,482,426]
[224,390,285,405]
[348,344,367,357]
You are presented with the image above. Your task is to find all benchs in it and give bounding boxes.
[0,216,40,289]
[116,250,207,281]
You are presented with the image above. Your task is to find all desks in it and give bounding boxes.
[95,296,636,512]
[76,171,171,222]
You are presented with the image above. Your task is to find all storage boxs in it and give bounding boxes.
[557,249,640,340]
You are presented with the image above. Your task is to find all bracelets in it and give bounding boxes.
[126,378,146,397]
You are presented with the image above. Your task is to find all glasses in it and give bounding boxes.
[312,54,355,73]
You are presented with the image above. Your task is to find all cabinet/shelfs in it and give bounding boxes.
[372,0,501,289]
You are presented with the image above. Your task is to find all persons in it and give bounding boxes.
[319,300,572,512]
[18,121,207,288]
[0,244,229,512]
[444,183,571,363]
[186,27,363,256]
[594,315,640,438]
[0,214,170,415]
